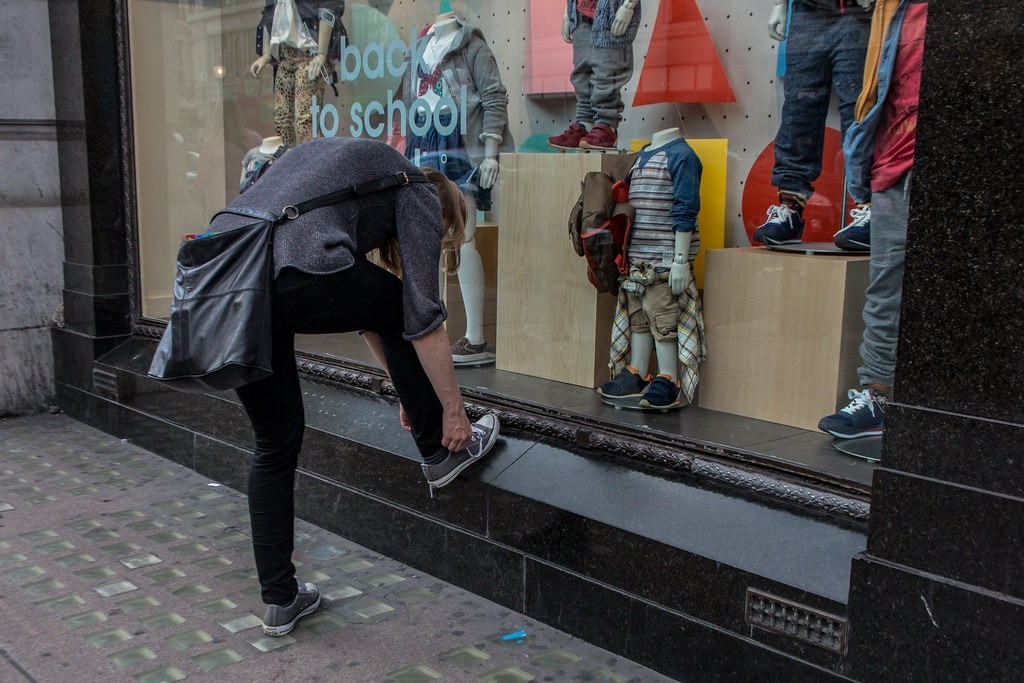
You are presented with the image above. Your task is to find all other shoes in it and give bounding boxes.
[450,337,490,363]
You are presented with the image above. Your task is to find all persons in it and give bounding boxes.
[752,1,873,250]
[817,1,930,442]
[545,0,641,152]
[237,136,290,193]
[188,137,504,636]
[249,1,349,147]
[596,127,704,411]
[405,11,506,362]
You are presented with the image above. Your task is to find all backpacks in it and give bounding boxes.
[570,170,636,297]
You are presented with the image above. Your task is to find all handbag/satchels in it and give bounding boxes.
[146,214,286,391]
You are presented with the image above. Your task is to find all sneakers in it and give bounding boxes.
[262,576,321,638]
[817,384,892,438]
[751,205,805,244]
[548,121,590,149]
[579,124,620,149]
[832,200,871,252]
[595,364,655,398]
[640,373,683,408]
[421,412,500,489]
